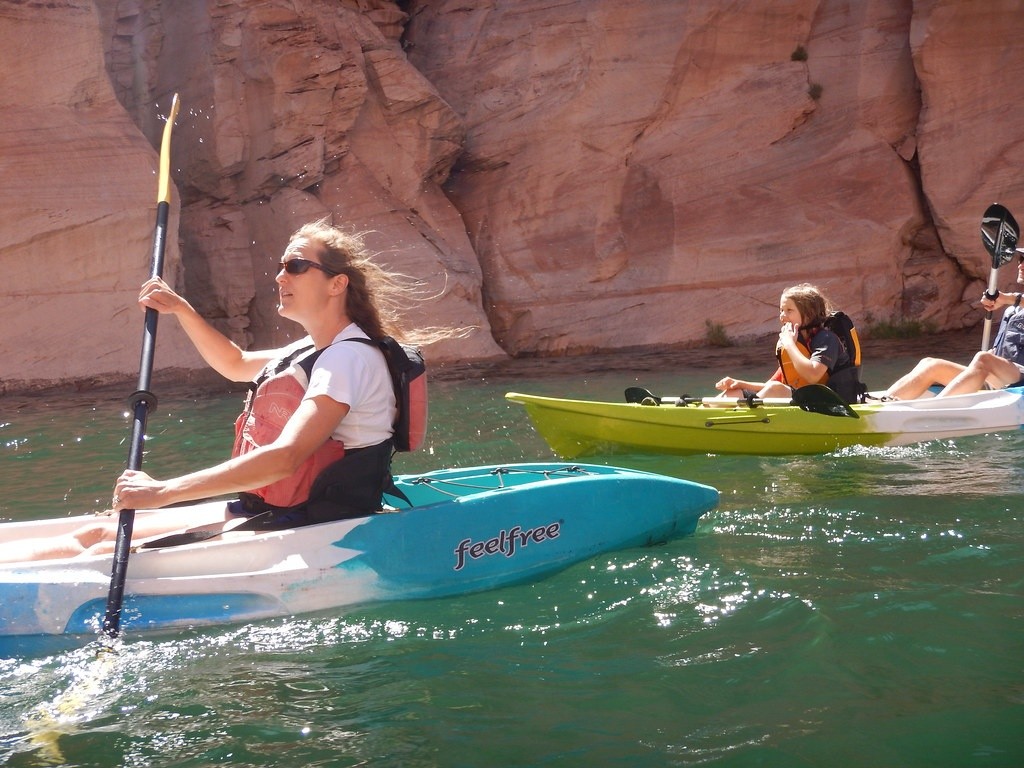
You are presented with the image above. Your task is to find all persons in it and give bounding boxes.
[879,248,1024,402]
[642,282,863,407]
[0,212,480,562]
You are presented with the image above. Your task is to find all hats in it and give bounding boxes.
[1015,247,1024,253]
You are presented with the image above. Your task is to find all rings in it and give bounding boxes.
[112,495,121,504]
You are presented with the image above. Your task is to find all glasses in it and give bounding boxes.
[1017,255,1024,265]
[277,258,352,289]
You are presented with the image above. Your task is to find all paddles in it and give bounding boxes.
[94,90,185,659]
[623,384,860,420]
[977,202,1019,351]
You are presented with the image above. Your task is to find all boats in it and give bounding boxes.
[0,460,723,641]
[508,378,1024,462]
